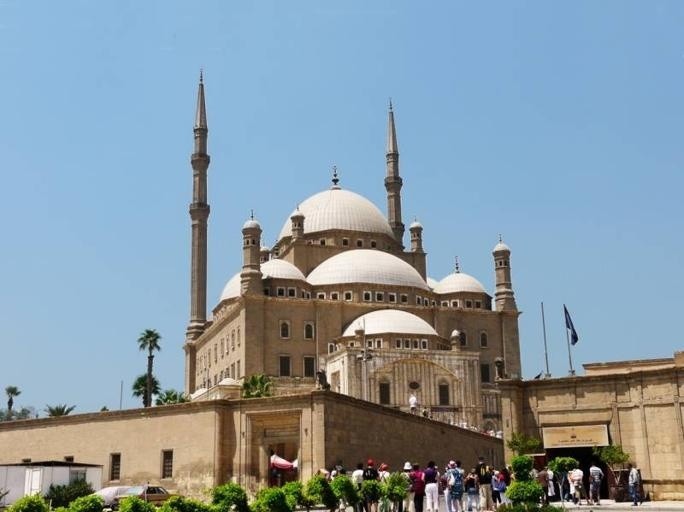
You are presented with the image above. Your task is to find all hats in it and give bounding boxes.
[404,461,413,470]
[448,461,457,468]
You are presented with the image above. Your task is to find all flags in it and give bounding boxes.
[563,304,579,346]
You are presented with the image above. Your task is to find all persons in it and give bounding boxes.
[626,462,644,506]
[466,423,503,440]
[270,466,280,487]
[422,406,428,418]
[313,454,605,511]
[283,465,295,483]
[408,393,420,414]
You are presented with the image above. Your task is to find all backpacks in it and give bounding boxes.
[451,472,462,498]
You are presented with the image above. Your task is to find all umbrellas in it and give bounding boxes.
[269,453,293,470]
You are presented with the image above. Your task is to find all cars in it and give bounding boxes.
[91,486,132,506]
[111,484,181,510]
